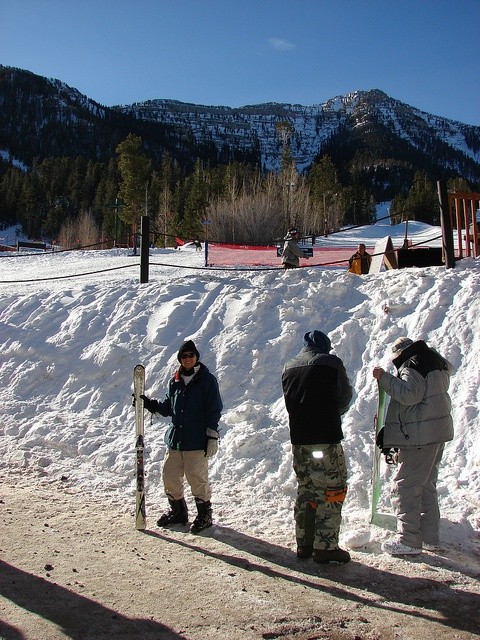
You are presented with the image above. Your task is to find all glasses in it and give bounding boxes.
[179,353,196,359]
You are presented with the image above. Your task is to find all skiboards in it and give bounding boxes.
[134,364,147,530]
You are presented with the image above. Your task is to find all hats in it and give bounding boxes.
[302,330,333,350]
[178,340,200,361]
[288,227,298,236]
[391,337,412,352]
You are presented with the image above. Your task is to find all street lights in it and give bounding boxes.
[285,182,289,229]
[291,183,294,228]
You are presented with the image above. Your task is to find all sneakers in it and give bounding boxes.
[297,547,313,558]
[313,548,350,563]
[422,541,440,550]
[381,539,422,555]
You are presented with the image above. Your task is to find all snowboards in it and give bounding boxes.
[367,379,387,524]
[277,247,314,257]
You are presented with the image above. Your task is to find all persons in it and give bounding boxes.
[130,339,224,533]
[348,243,373,274]
[280,227,309,269]
[280,328,353,565]
[371,336,455,555]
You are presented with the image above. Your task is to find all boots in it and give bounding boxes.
[190,497,212,532]
[156,493,188,527]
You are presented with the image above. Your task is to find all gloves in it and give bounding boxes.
[204,428,222,457]
[131,393,158,413]
